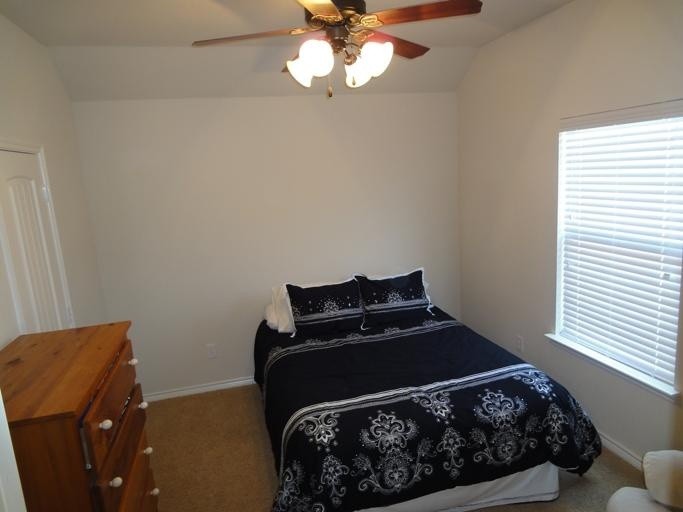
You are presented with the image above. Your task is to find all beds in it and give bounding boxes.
[253,307,561,512]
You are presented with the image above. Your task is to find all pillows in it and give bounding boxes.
[266,268,434,338]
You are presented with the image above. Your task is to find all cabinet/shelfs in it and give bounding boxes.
[0,322,159,512]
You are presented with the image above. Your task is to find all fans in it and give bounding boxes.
[191,1,485,59]
